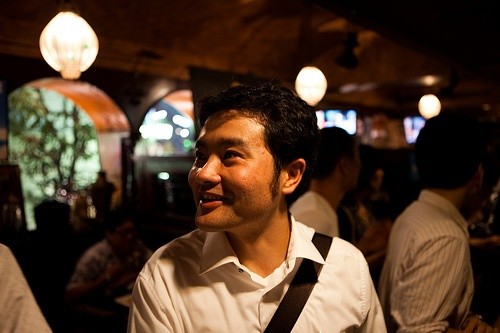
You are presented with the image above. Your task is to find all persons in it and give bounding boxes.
[0,194,152,333]
[379,114,499,333]
[127,80,387,333]
[288,126,420,289]
[91,172,117,224]
